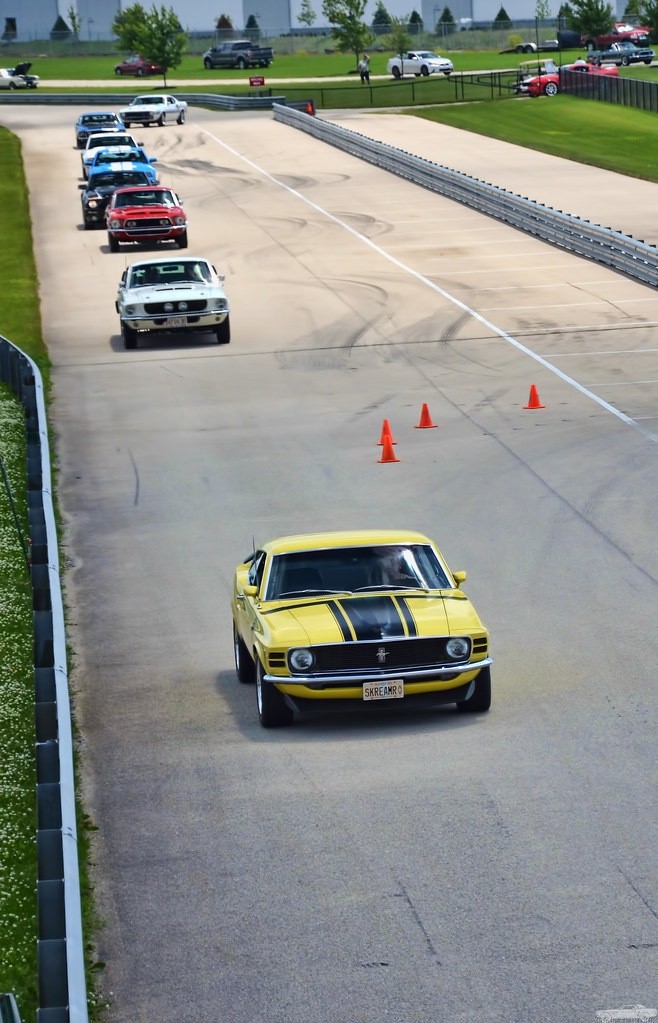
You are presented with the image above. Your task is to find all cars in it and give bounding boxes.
[387,51,453,78]
[231,529,492,728]
[114,54,164,76]
[115,257,231,350]
[84,147,157,166]
[78,162,159,229]
[81,132,144,180]
[0,62,40,90]
[104,186,188,252]
[558,30,586,48]
[594,22,649,51]
[119,94,188,129]
[76,111,125,149]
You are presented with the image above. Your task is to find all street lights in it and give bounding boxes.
[433,9,441,28]
[557,14,563,66]
[535,16,540,67]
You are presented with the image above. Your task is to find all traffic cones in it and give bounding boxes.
[377,435,400,463]
[414,403,438,427]
[377,419,397,445]
[522,384,545,408]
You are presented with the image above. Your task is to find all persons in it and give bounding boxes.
[358,54,370,85]
[575,56,599,67]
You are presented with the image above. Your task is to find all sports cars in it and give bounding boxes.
[520,64,619,96]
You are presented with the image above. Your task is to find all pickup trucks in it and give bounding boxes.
[587,43,654,66]
[203,40,273,70]
